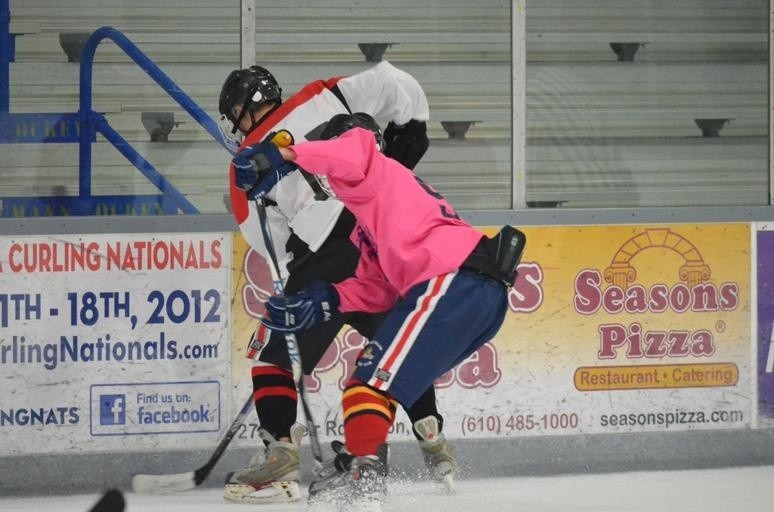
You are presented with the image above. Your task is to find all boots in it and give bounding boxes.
[225,422,306,486]
[411,416,454,478]
[309,439,390,495]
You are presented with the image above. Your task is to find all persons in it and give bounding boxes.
[218,60,456,488]
[230,111,508,496]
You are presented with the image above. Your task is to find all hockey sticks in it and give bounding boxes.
[130,392,254,494]
[247,158,327,486]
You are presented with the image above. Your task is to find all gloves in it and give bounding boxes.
[259,278,340,336]
[232,142,296,200]
[383,119,429,170]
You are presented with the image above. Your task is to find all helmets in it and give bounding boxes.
[218,65,281,154]
[315,112,381,200]
